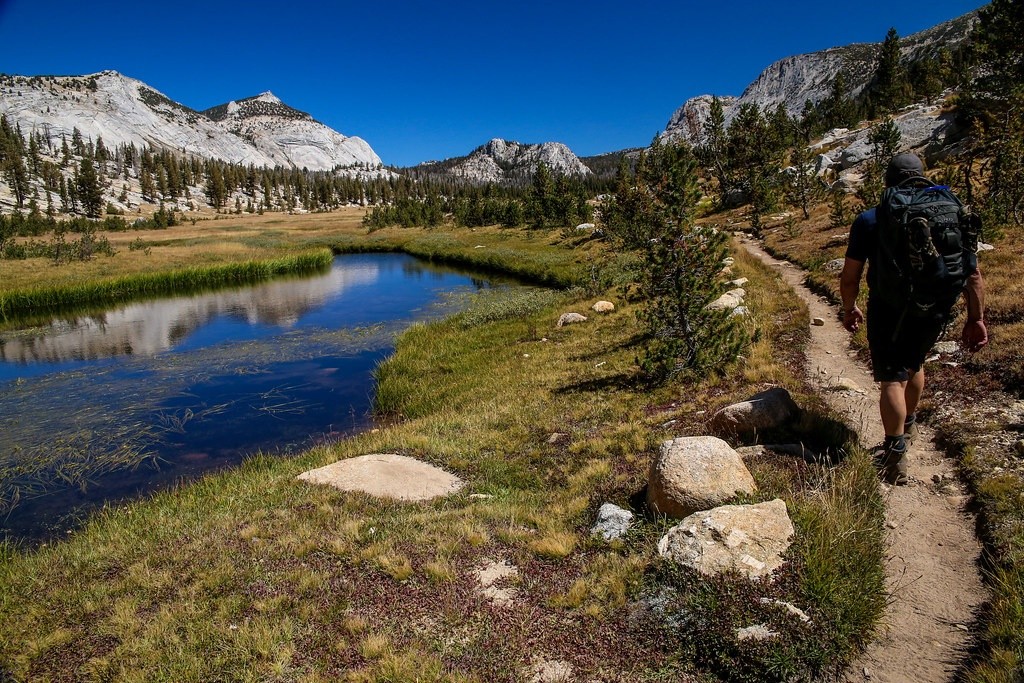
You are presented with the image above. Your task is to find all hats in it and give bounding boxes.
[886,154,923,178]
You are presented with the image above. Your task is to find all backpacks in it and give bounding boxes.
[876,176,982,318]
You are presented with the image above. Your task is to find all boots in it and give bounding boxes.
[874,442,908,484]
[903,413,918,451]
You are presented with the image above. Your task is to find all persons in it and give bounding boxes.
[839,152,988,485]
